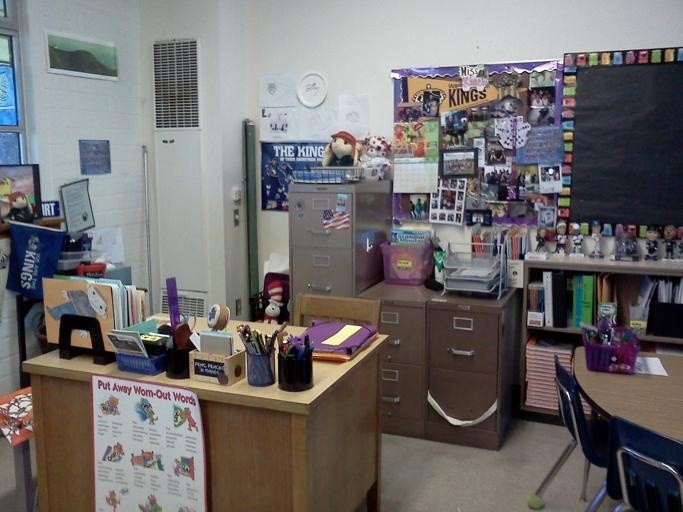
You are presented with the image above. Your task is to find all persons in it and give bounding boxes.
[588,220,603,255]
[663,224,676,259]
[553,221,568,255]
[676,226,683,256]
[409,88,559,220]
[644,226,660,262]
[570,222,583,254]
[534,227,547,253]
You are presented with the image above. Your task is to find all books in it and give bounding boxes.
[41,273,147,352]
[657,276,683,304]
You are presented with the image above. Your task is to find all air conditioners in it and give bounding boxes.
[150,38,222,316]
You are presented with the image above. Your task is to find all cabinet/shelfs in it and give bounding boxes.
[516,250,681,421]
[286,181,392,326]
[426,288,523,451]
[357,280,440,441]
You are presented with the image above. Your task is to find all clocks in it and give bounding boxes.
[297,73,329,109]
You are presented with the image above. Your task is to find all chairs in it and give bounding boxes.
[528,353,682,511]
[292,294,381,331]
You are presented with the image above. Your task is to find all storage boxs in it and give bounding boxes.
[438,242,512,301]
[469,223,530,290]
[378,237,435,286]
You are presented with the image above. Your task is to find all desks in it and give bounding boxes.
[21,312,389,512]
[574,345,683,446]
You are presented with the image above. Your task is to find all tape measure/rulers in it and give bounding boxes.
[167,277,180,341]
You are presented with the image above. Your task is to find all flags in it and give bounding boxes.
[323,209,350,229]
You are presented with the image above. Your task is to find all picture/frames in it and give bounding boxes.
[75,136,116,179]
[40,29,121,84]
[391,191,431,224]
[465,200,509,227]
[429,180,467,227]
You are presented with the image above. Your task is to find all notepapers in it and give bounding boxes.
[199,331,234,356]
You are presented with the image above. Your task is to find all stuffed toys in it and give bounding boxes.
[8,191,36,223]
[260,280,284,326]
[321,130,392,180]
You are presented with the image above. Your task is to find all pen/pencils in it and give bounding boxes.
[238,321,314,386]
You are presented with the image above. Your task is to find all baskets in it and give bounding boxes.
[287,167,364,184]
[584,324,641,375]
[115,351,166,376]
